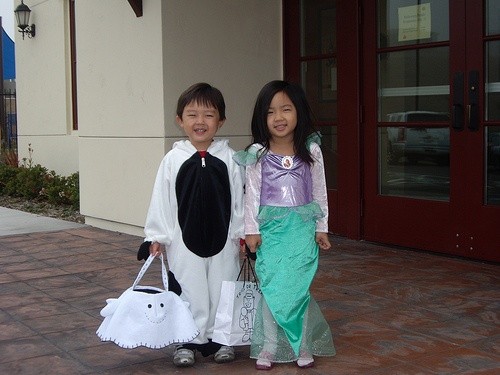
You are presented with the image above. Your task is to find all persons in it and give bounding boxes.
[232,80,337,370]
[144,82,246,366]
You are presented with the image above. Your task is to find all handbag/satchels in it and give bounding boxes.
[96,251,201,349]
[211,251,264,347]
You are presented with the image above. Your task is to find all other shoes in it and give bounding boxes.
[297,349,314,367]
[171,346,196,367]
[255,351,273,370]
[213,345,236,363]
